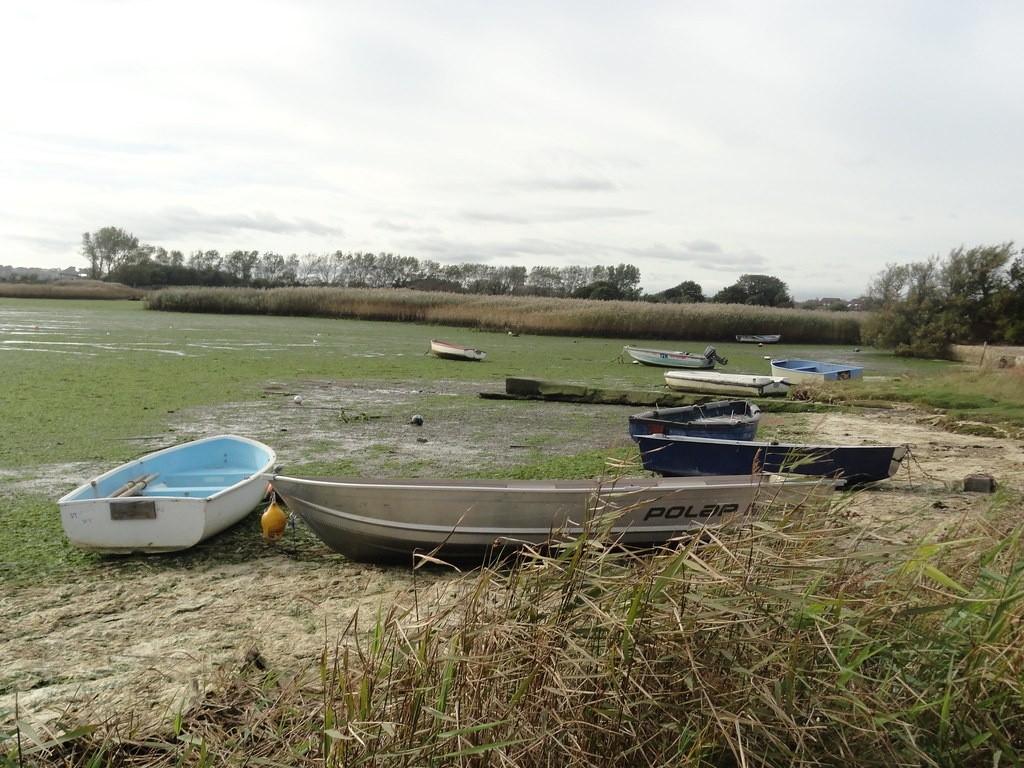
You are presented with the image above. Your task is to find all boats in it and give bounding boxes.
[57,434,278,558]
[770,358,864,386]
[633,430,917,492]
[736,334,781,344]
[260,470,848,574]
[623,345,716,370]
[627,399,762,442]
[664,370,791,399]
[430,338,487,362]
